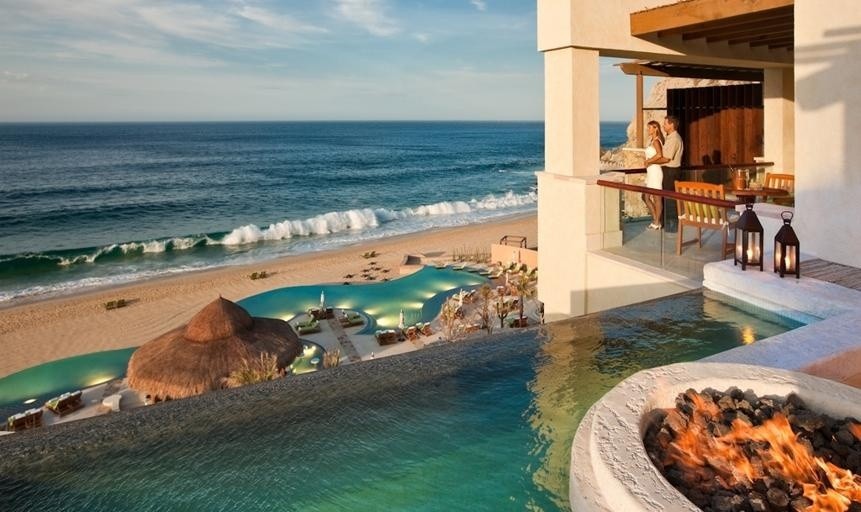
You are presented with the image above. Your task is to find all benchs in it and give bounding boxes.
[676,180,738,262]
[763,172,795,206]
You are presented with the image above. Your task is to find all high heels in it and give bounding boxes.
[647,223,662,231]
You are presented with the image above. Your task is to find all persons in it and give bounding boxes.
[646,114,684,234]
[640,120,666,231]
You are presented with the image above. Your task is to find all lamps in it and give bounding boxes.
[775,206,801,279]
[735,203,767,274]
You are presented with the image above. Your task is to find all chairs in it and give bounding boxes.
[293,306,365,334]
[5,389,83,435]
[368,248,542,347]
